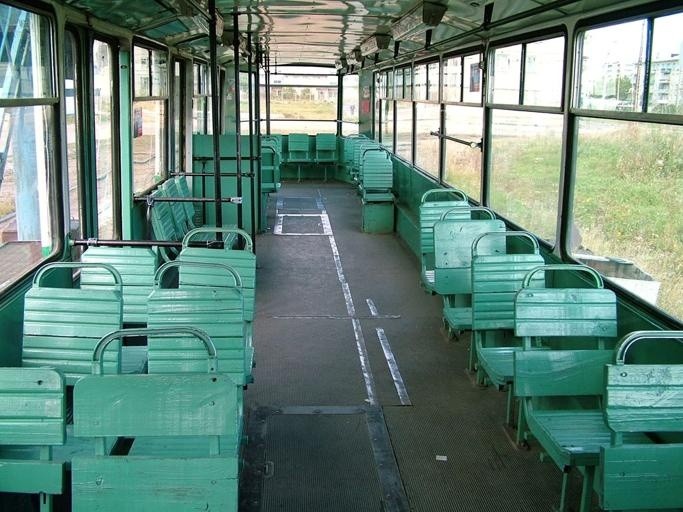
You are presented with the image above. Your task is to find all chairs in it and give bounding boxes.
[286,133,312,184]
[314,133,337,183]
